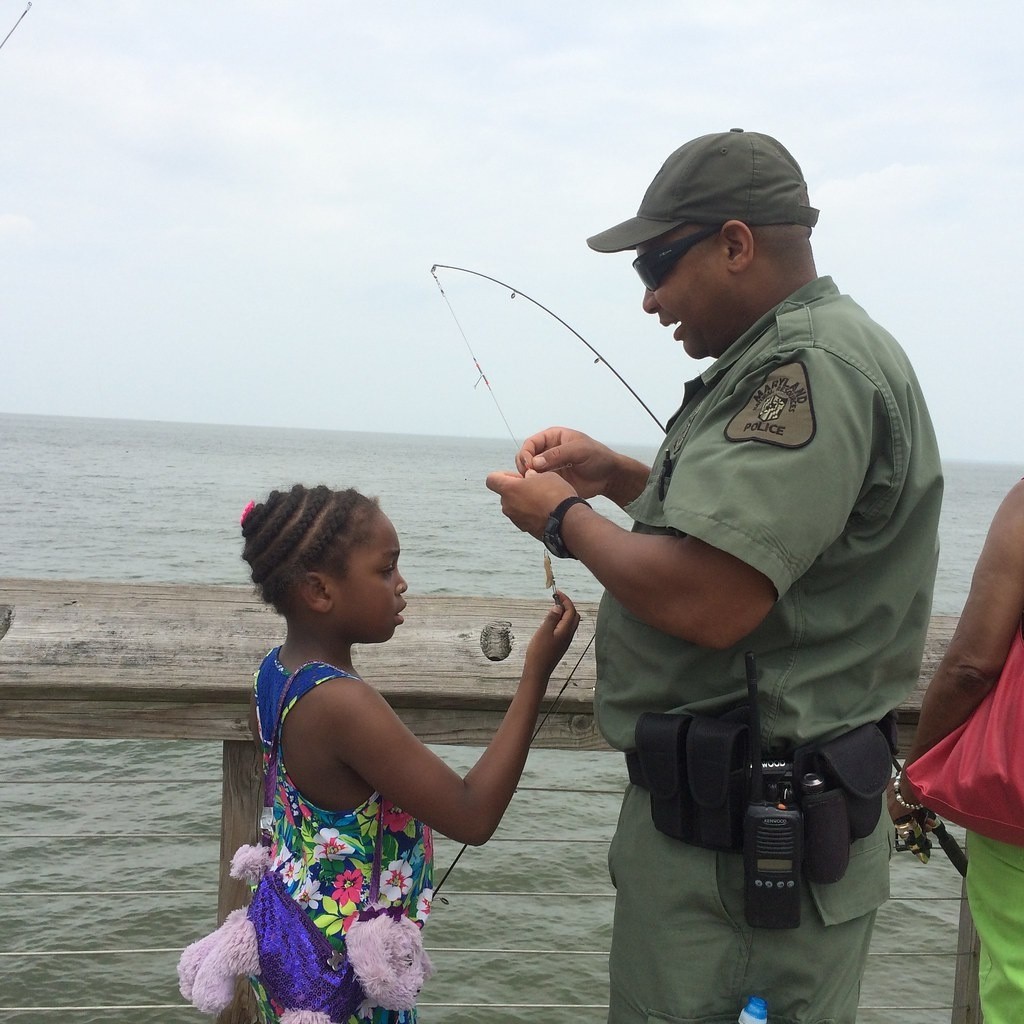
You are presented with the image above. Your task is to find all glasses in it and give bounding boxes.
[630,224,718,291]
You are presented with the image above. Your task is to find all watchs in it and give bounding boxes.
[542,496,592,561]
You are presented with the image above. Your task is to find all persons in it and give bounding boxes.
[486,130,945,1024]
[240,484,581,1024]
[885,478,1024,1024]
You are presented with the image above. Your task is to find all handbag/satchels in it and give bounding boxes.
[174,835,433,1022]
[906,631,1024,846]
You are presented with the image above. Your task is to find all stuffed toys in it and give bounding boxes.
[178,844,425,1024]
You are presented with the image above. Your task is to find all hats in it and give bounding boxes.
[582,126,823,253]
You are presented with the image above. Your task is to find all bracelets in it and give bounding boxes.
[894,771,923,810]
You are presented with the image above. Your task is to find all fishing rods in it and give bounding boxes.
[426,258,970,880]
[431,634,597,896]
